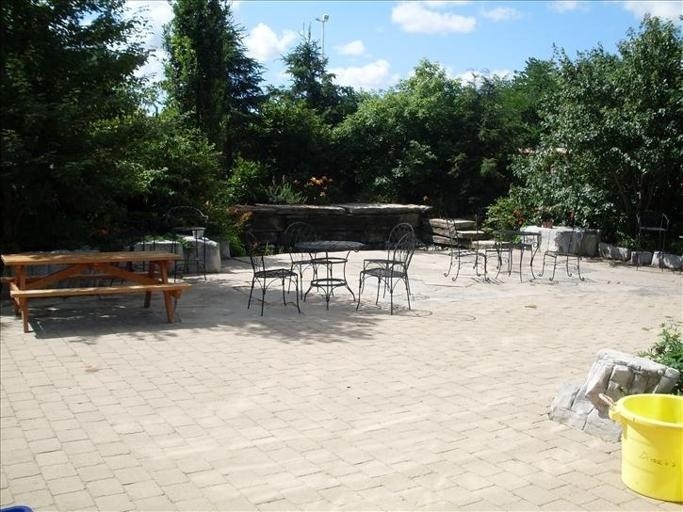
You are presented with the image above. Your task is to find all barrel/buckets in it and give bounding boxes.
[597,391,683,504]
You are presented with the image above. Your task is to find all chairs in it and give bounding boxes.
[168,206,210,280]
[636,209,670,252]
[245,223,416,316]
[444,213,588,282]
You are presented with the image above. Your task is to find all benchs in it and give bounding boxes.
[0,269,161,280]
[10,282,194,298]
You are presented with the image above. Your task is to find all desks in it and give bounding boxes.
[0,251,183,333]
